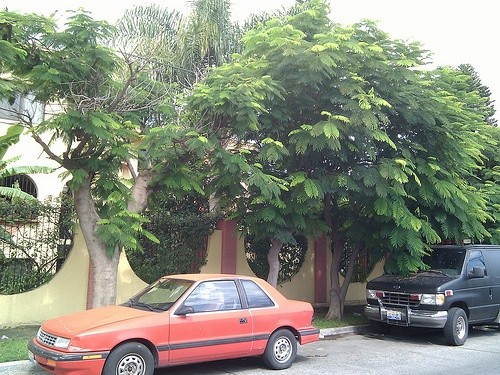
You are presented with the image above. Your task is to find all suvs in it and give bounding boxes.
[365,245,500,345]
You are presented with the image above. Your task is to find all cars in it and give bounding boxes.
[28,273,320,375]
